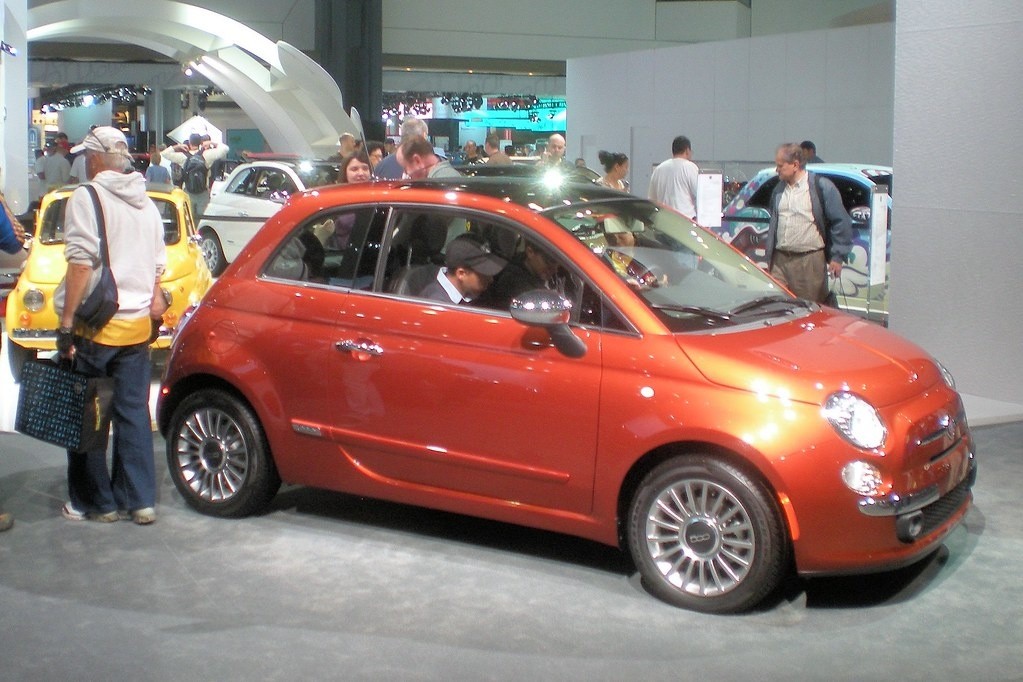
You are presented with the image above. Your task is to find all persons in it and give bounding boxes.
[647,135,708,225]
[33,124,88,206]
[418,216,697,312]
[597,150,632,202]
[324,117,586,253]
[798,141,825,165]
[145,133,230,229]
[56,126,166,524]
[263,174,282,199]
[768,141,852,303]
[0,193,26,532]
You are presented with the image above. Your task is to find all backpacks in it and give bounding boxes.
[181,149,209,193]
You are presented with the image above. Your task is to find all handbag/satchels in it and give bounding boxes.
[824,275,849,315]
[75,265,119,332]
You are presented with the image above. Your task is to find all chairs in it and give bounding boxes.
[299,232,325,271]
[485,220,524,260]
[384,215,447,299]
[267,174,282,190]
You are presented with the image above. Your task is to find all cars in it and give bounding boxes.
[712,163,894,323]
[428,153,542,179]
[123,152,151,176]
[154,174,979,618]
[194,159,343,279]
[4,182,214,383]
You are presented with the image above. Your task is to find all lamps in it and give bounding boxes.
[179,65,224,112]
[382,91,557,124]
[0,40,17,58]
[39,80,155,115]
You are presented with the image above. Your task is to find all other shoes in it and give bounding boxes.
[0,513,14,531]
[118,507,156,524]
[61,501,119,522]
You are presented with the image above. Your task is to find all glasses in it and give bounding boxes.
[400,161,414,174]
[88,124,108,152]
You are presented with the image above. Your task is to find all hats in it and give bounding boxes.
[54,133,67,140]
[45,139,57,146]
[69,126,128,155]
[444,232,508,276]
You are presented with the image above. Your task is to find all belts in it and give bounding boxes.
[778,249,820,257]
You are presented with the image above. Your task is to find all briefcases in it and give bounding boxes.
[13,353,116,456]
